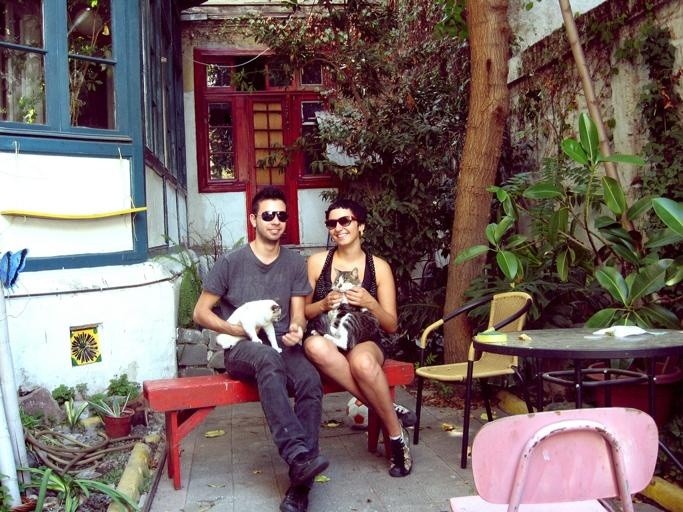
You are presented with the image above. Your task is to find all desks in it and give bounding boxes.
[470,326,683,412]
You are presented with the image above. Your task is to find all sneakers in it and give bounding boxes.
[289,451,329,485]
[279,485,309,511]
[392,404,418,427]
[389,422,412,478]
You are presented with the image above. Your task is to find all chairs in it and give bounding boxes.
[448,407,659,512]
[413,292,533,469]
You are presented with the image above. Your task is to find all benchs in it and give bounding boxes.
[144,358,415,489]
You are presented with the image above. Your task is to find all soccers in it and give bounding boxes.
[346,397,368,429]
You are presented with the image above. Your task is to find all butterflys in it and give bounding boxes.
[0,248,27,298]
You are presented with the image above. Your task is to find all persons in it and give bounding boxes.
[193,187,329,512]
[303,199,418,478]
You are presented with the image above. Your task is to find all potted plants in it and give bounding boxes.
[86,386,134,439]
[455,113,683,426]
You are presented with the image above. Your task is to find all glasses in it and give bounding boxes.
[325,216,358,230]
[253,210,289,223]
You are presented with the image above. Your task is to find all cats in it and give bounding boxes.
[215,299,284,355]
[310,267,370,350]
